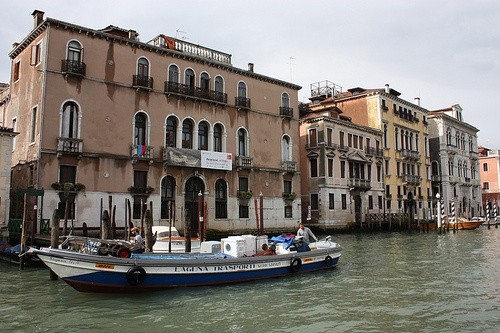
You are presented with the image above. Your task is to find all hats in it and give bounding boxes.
[131,227,139,231]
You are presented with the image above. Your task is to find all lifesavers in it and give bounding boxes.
[325,256,333,267]
[126,265,147,287]
[290,257,302,272]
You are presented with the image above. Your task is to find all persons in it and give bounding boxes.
[297,222,317,245]
[128,227,144,253]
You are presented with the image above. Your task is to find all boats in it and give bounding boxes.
[420,216,485,230]
[32,241,343,293]
[151,224,201,253]
